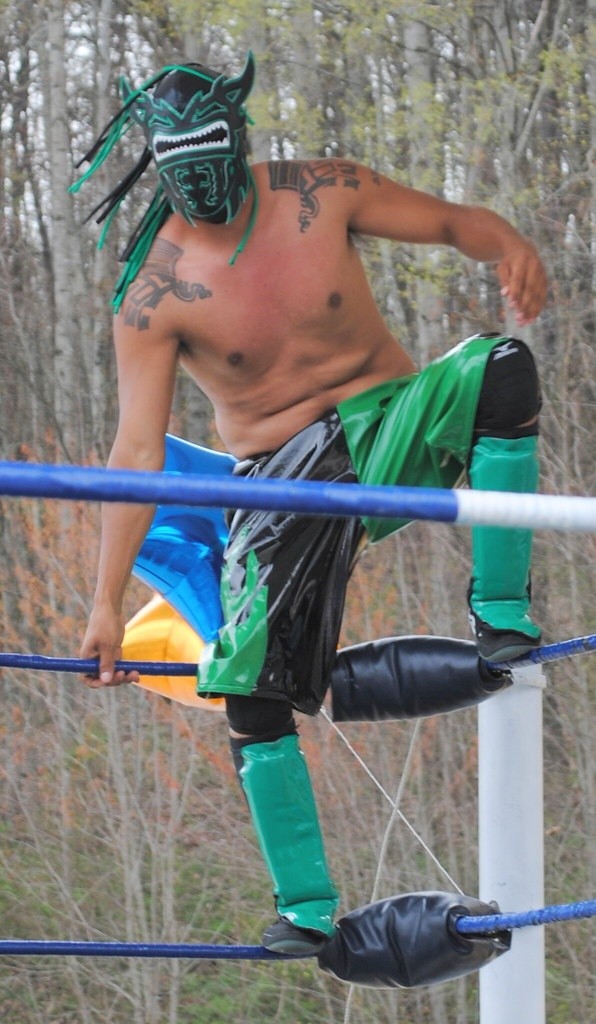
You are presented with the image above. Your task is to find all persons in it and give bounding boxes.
[75,50,547,957]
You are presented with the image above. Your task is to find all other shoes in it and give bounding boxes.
[262,917,325,954]
[467,601,542,661]
